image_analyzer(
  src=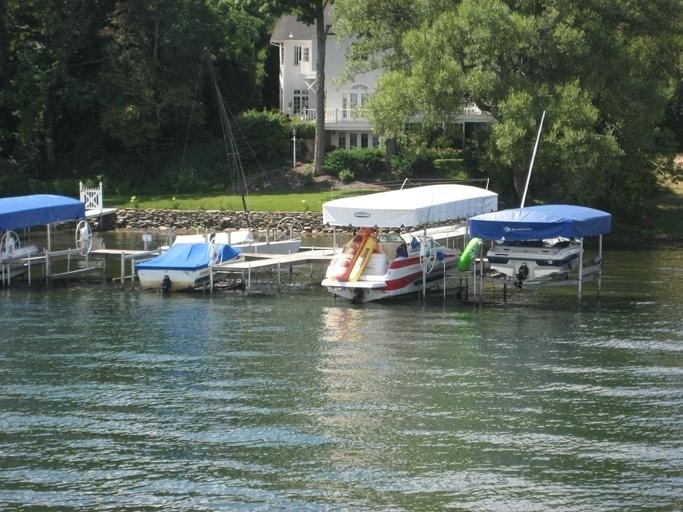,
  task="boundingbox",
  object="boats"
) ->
[321,184,613,305]
[134,228,302,292]
[0,194,97,286]
